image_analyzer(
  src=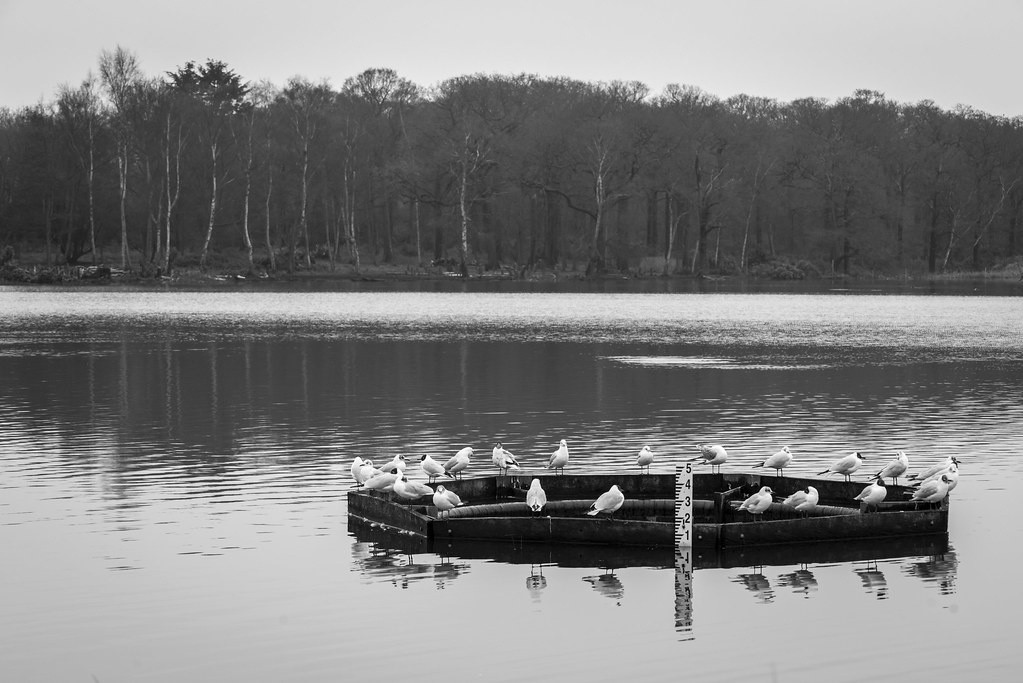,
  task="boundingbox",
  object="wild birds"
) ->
[526,478,548,517]
[526,563,624,608]
[688,444,727,474]
[912,462,959,491]
[868,451,909,485]
[783,486,819,518]
[350,457,464,519]
[905,456,962,481]
[852,479,887,512]
[443,447,474,480]
[379,454,452,483]
[817,452,866,482]
[492,441,519,475]
[587,485,625,520]
[545,438,569,475]
[635,445,653,475]
[752,446,793,477]
[731,552,954,604]
[737,486,776,523]
[909,474,950,510]
